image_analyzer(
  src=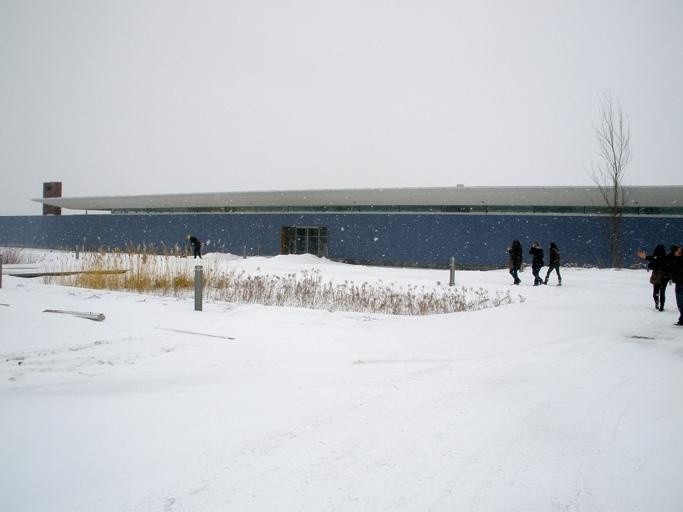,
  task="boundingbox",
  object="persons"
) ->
[637,243,682,326]
[529,241,545,286]
[648,243,672,311]
[186,233,202,260]
[542,241,562,286]
[507,239,523,284]
